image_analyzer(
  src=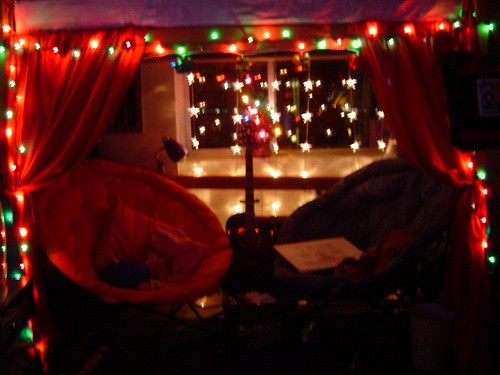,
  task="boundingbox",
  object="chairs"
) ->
[34,158,237,357]
[274,157,461,355]
